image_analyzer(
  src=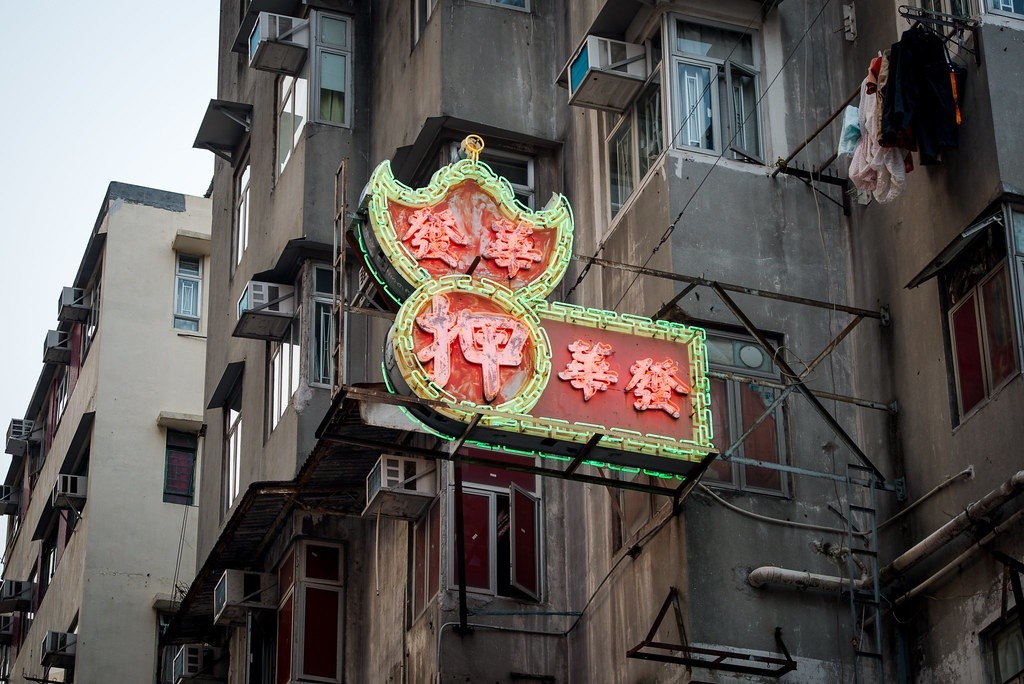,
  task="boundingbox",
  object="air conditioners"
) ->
[236,281,295,338]
[5,418,33,457]
[0,485,19,515]
[567,34,644,112]
[40,630,77,667]
[43,330,72,365]
[58,286,90,322]
[52,474,87,510]
[0,580,31,599]
[249,12,309,74]
[213,569,276,626]
[360,454,437,520]
[172,643,226,684]
[0,615,14,638]
